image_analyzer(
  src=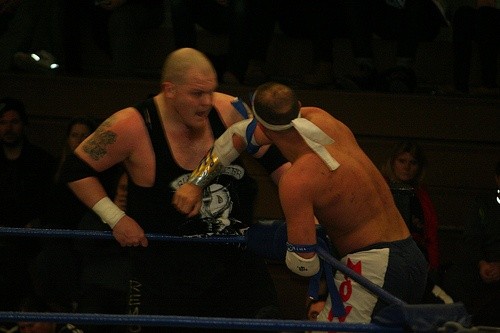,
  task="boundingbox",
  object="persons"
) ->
[0,104,110,276]
[57,48,291,333]
[6,295,83,333]
[173,82,426,333]
[459,159,500,328]
[0,0,500,96]
[380,142,439,279]
